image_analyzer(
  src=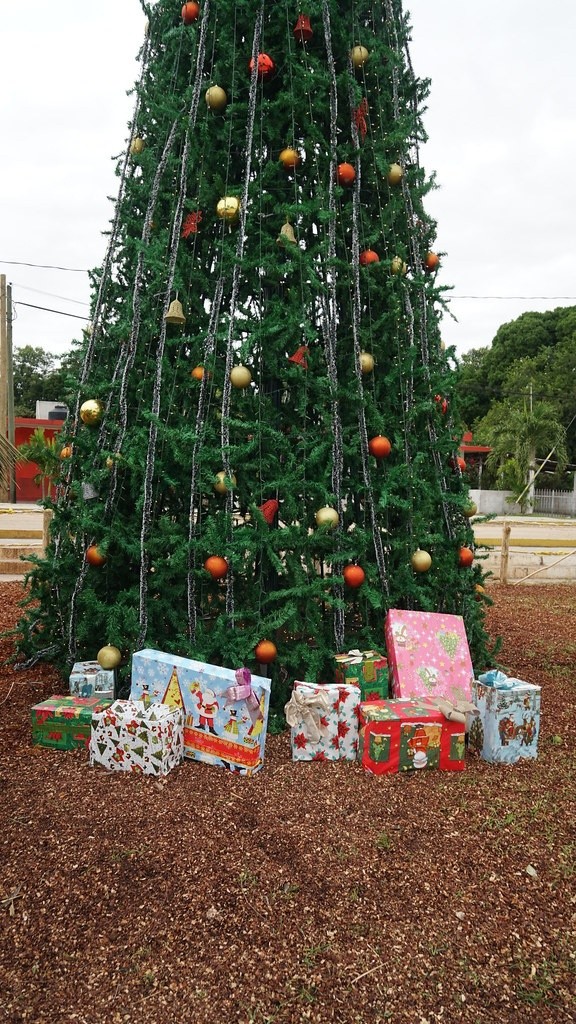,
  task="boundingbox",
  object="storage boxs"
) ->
[283,680,361,761]
[333,651,389,701]
[359,697,466,774]
[131,648,271,776]
[468,670,541,764]
[89,699,184,776]
[69,661,117,699]
[384,609,475,733]
[30,695,116,750]
